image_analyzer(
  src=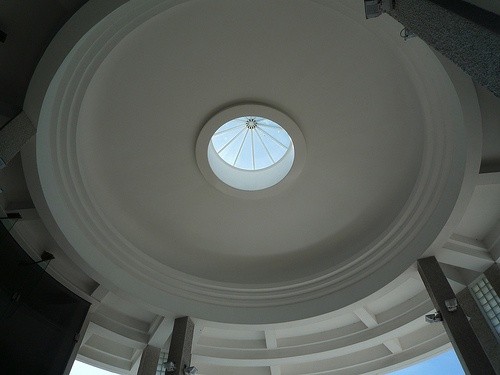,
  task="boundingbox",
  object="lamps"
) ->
[183,366,199,375]
[425,311,443,323]
[163,361,176,372]
[401,26,417,41]
[445,298,458,312]
[363,1,384,20]
